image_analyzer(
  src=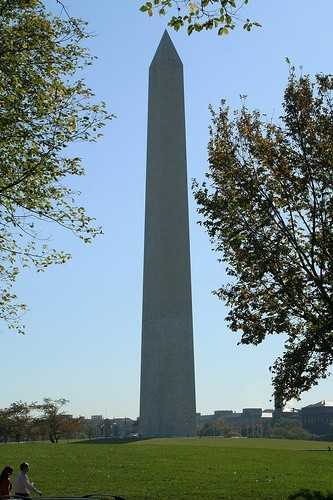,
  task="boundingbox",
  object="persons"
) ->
[0,465,13,500]
[14,462,42,499]
